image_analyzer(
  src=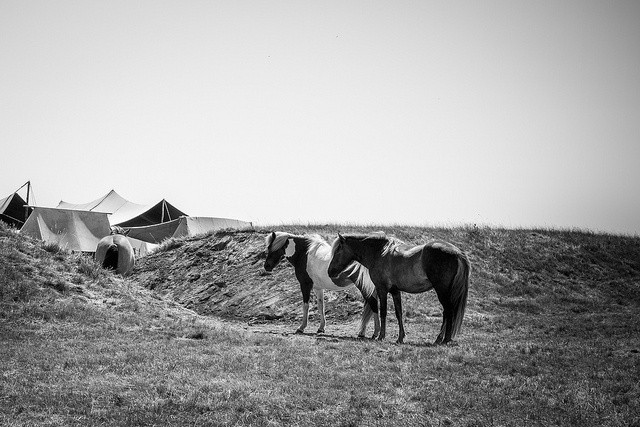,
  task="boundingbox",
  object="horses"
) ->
[326,231,473,346]
[263,231,382,341]
[95,225,136,278]
